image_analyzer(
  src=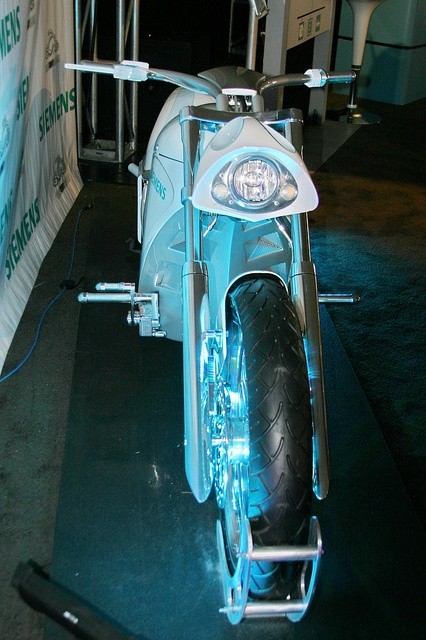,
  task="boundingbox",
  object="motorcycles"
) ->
[64,60,359,622]
